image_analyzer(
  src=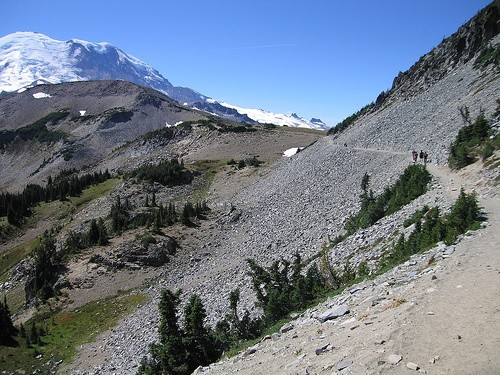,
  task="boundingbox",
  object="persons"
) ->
[411,149,428,163]
